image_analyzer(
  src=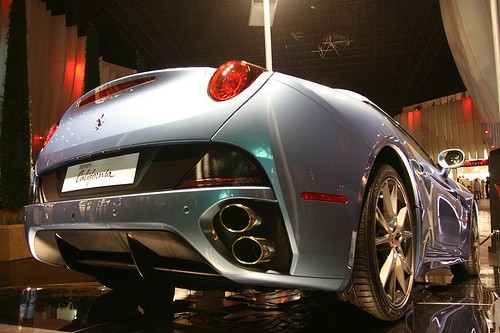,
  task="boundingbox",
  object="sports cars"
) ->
[22,60,480,322]
